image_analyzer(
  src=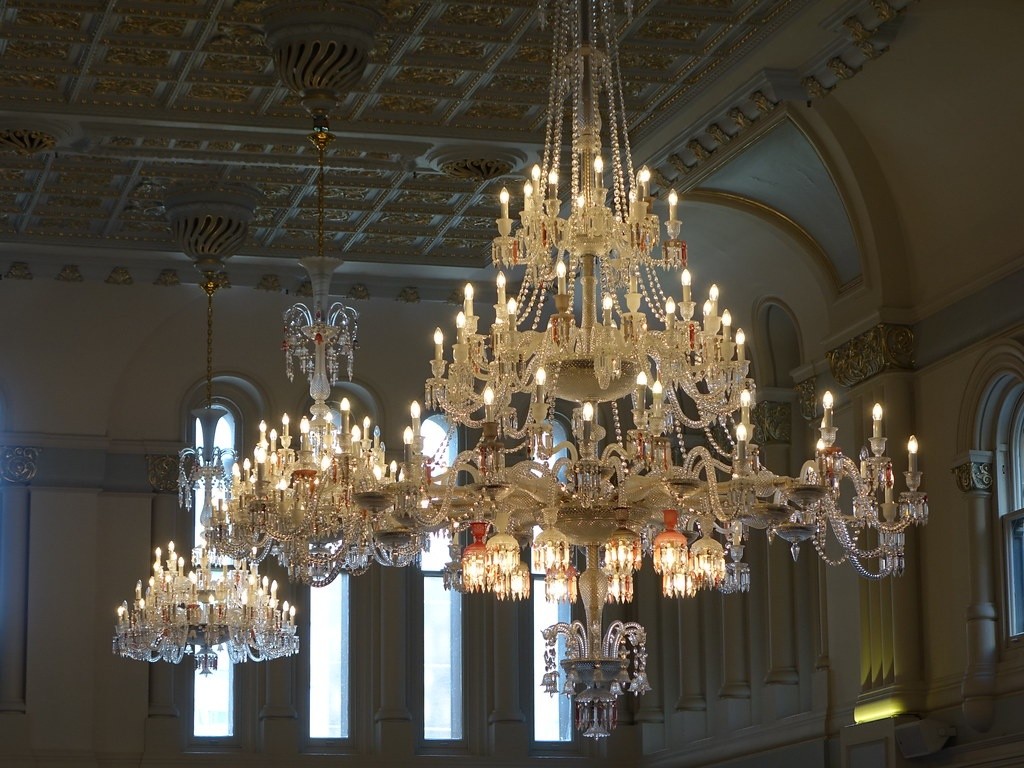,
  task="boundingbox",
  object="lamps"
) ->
[204,110,431,587]
[401,0,928,606]
[540,547,652,741]
[894,719,956,759]
[112,272,300,678]
